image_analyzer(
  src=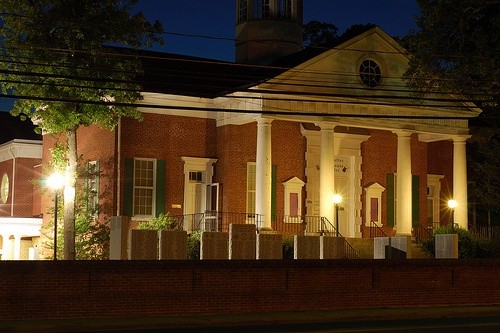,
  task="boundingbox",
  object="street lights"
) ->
[48,171,63,260]
[333,194,342,237]
[448,200,457,229]
